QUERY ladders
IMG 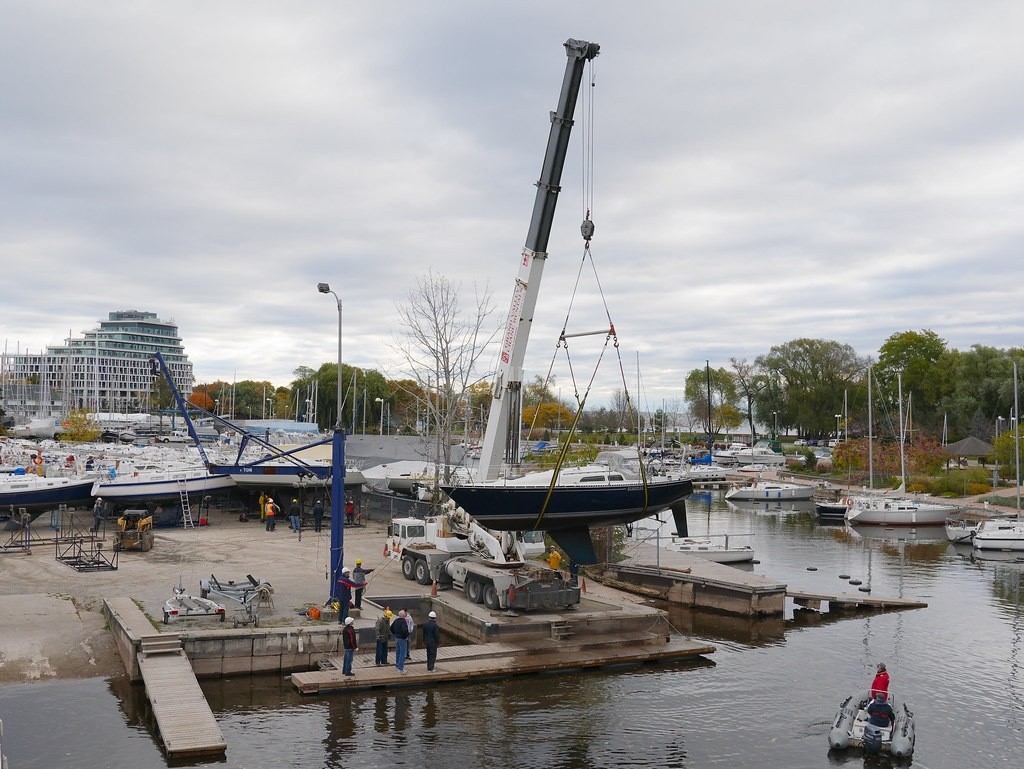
[177,476,194,530]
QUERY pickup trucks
[159,431,194,443]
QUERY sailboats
[815,355,1024,551]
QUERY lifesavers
[77,464,83,473]
[959,521,964,529]
[34,455,43,464]
[847,499,853,505]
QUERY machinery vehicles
[385,39,648,613]
[112,510,155,552]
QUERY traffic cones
[582,577,588,596]
[429,578,440,598]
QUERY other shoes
[406,657,412,660]
[381,661,390,664]
[342,671,345,674]
[345,672,355,676]
[376,662,381,666]
[428,669,437,673]
[395,667,406,675]
[342,622,344,625]
[338,621,341,624]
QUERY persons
[375,610,393,666]
[423,610,440,673]
[336,567,367,628]
[867,693,895,728]
[352,558,375,610]
[342,617,359,676]
[345,498,358,523]
[389,609,409,672]
[265,427,272,443]
[93,497,104,531]
[287,499,302,533]
[224,433,231,445]
[265,498,281,531]
[870,663,889,702]
[401,608,414,661]
[86,456,94,471]
[313,500,325,532]
[259,490,268,523]
[544,545,562,571]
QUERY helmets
[293,499,297,503]
[98,498,102,501]
[385,610,393,618]
[269,499,273,502]
[345,617,354,625]
[342,567,349,573]
[429,611,436,618]
[317,501,321,503]
[356,559,362,564]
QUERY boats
[630,351,786,480]
[410,434,693,528]
[828,689,916,757]
[0,329,475,513]
[637,532,756,562]
[725,480,815,500]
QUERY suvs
[794,438,844,448]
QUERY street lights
[835,414,841,444]
[318,283,342,429]
[266,398,271,420]
[285,406,288,420]
[375,398,383,436]
[999,418,1005,439]
[774,411,777,439]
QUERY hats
[876,693,884,698]
[549,546,556,549]
